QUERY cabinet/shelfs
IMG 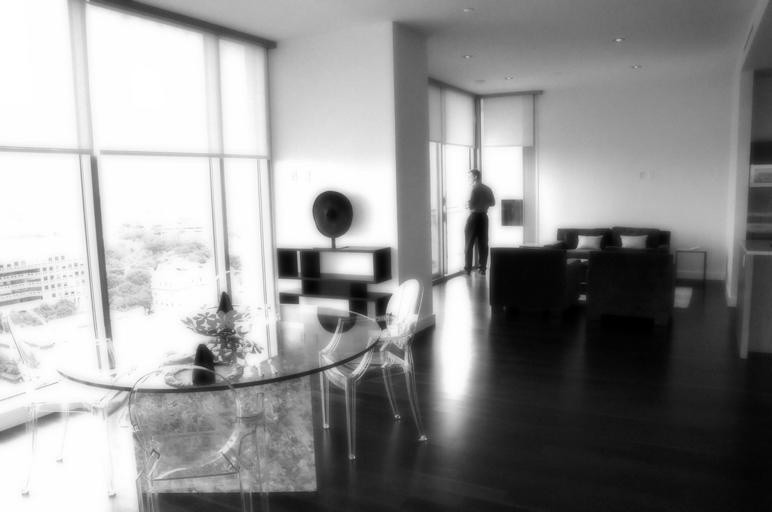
[276,246,392,334]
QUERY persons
[460,168,496,275]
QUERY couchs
[543,228,672,258]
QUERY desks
[674,247,708,302]
[737,238,772,360]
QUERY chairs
[0,280,427,511]
[490,247,673,325]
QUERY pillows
[575,235,648,252]
